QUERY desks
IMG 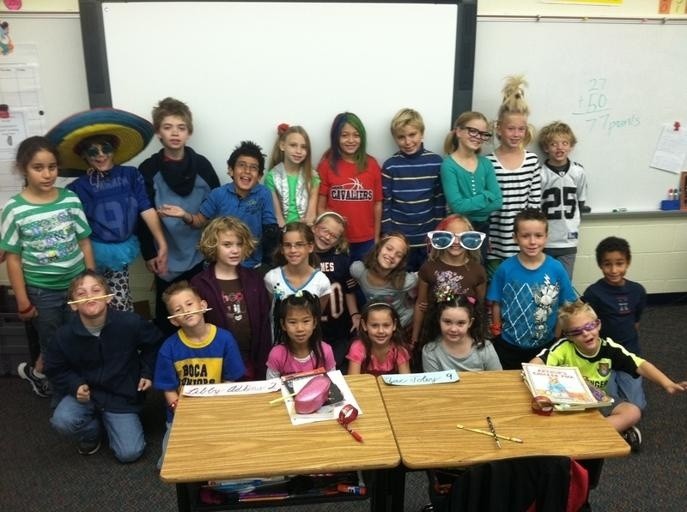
[375,361,633,512]
[155,369,405,511]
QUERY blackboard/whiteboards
[471,15,687,220]
[0,10,92,224]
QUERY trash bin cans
[0,314,30,377]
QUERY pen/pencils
[612,207,628,213]
[457,416,524,449]
[668,188,682,200]
[239,485,366,502]
[167,308,214,319]
[67,294,116,304]
[270,391,336,404]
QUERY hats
[43,107,154,177]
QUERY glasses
[316,223,337,240]
[460,126,492,141]
[427,230,487,251]
[86,145,114,158]
[563,319,600,337]
[279,241,311,250]
[234,161,260,171]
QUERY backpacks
[422,454,597,512]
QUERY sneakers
[621,425,642,452]
[17,361,54,398]
[76,433,102,456]
